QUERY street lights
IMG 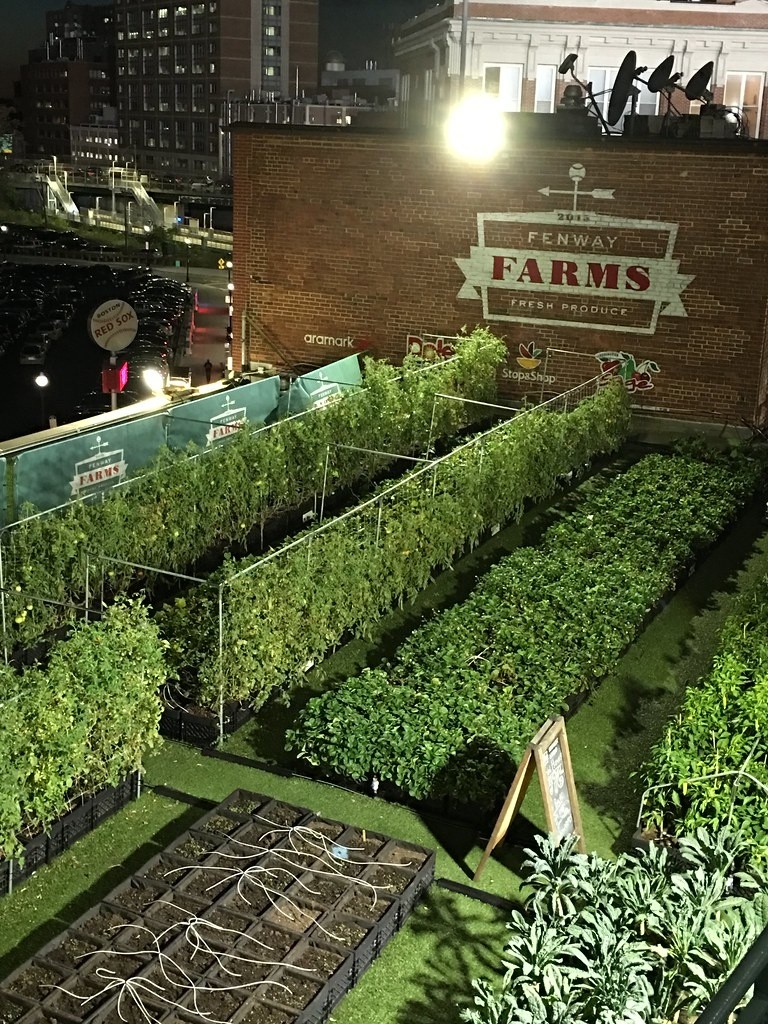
[51,155,57,175]
[96,197,103,218]
[227,283,234,305]
[225,260,233,282]
[209,207,216,230]
[35,372,49,431]
[228,306,234,327]
[125,161,131,191]
[127,201,134,225]
[203,212,210,229]
[112,160,119,189]
[174,201,182,225]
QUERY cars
[0,220,195,429]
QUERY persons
[216,362,224,378]
[203,359,212,384]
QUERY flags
[0,134,12,153]
